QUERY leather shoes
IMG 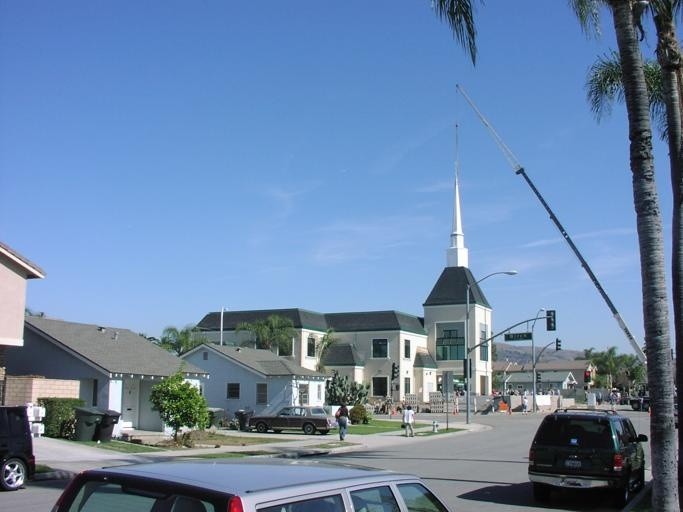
[406,435,414,437]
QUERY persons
[523,396,529,415]
[611,387,620,411]
[403,406,415,436]
[336,402,351,441]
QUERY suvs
[50,460,448,512]
[526,407,647,505]
[0,405,36,489]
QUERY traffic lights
[555,338,560,351]
[583,371,590,383]
[391,362,398,380]
[535,372,541,384]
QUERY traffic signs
[504,333,531,341]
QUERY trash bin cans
[93,410,122,442]
[235,411,255,432]
[74,407,105,441]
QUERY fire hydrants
[431,421,439,434]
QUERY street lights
[502,361,516,395]
[464,270,517,425]
[531,308,545,414]
[219,305,230,345]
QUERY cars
[589,388,629,406]
[248,406,337,435]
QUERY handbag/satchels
[401,424,406,428]
[335,410,341,417]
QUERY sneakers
[340,435,344,440]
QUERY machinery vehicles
[629,380,678,413]
